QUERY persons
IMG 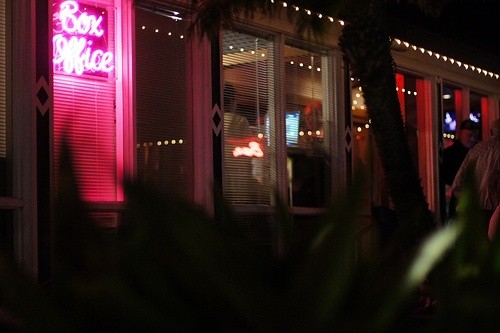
[221,80,256,203]
[439,119,483,218]
[444,119,500,220]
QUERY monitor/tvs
[264,109,312,149]
